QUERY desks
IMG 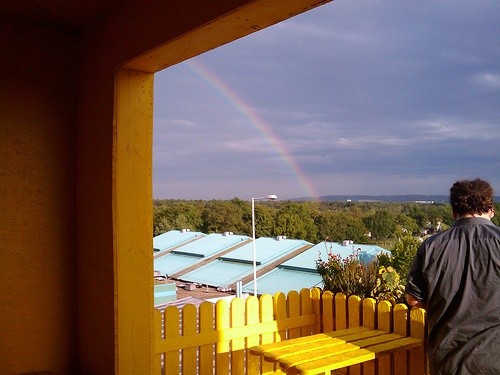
[250,324,423,375]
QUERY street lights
[251,194,277,297]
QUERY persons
[403,176,500,375]
